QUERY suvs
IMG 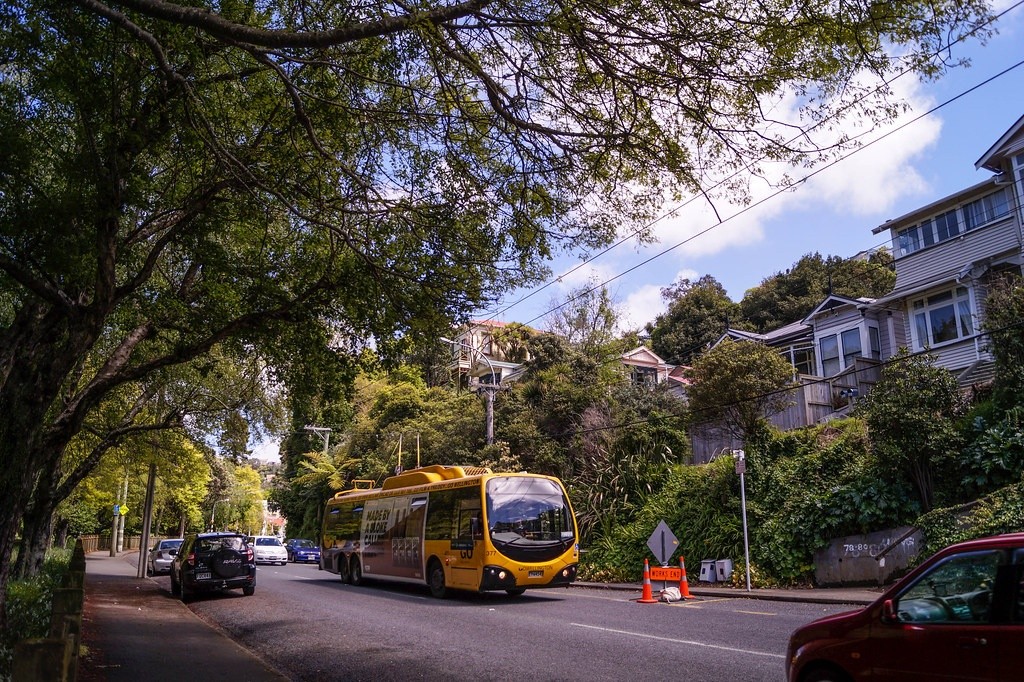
[170,531,256,601]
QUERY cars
[245,535,288,567]
[785,532,1024,682]
[148,538,188,575]
[285,539,320,564]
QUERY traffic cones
[637,558,659,603]
[675,556,695,598]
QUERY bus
[319,465,580,598]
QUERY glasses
[479,516,481,519]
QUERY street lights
[211,499,229,531]
[439,337,495,465]
